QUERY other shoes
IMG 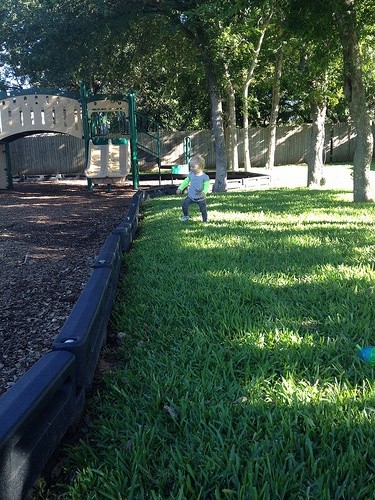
[181,215,189,221]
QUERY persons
[175,155,211,226]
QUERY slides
[82,138,132,183]
